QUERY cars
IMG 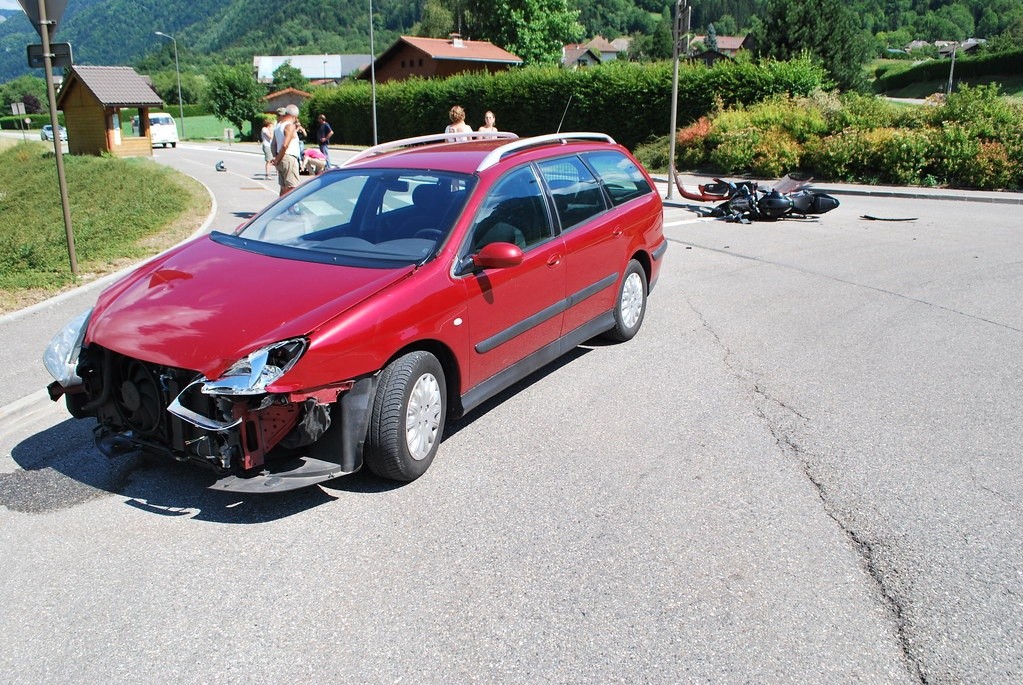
[41,128,667,493]
[41,125,69,144]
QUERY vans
[132,111,181,151]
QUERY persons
[445,106,473,191]
[261,104,334,216]
[476,111,497,140]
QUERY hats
[276,107,286,116]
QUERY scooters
[685,170,841,224]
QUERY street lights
[153,30,185,136]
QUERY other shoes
[266,174,273,180]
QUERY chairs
[469,184,526,254]
[394,184,454,239]
